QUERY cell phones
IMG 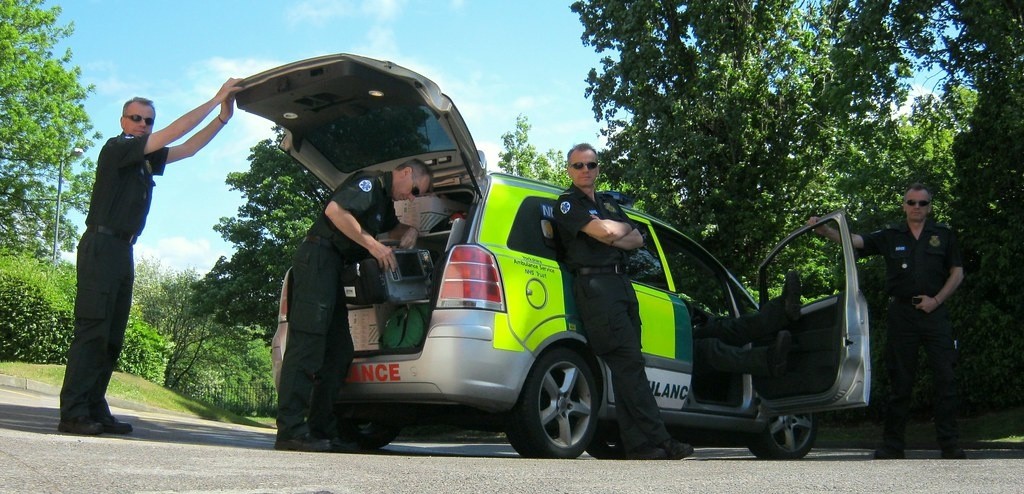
[912,297,922,305]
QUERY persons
[553,143,694,461]
[680,270,802,379]
[807,182,967,460]
[275,158,434,453]
[58,78,243,437]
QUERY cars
[230,52,870,461]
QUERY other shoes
[767,330,791,377]
[627,442,665,460]
[661,440,694,460]
[941,447,966,458]
[775,272,801,320]
[874,446,905,459]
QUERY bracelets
[408,226,420,234]
[609,240,616,248]
[218,114,228,125]
[935,296,941,307]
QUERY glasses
[411,177,420,197]
[906,200,929,206]
[124,115,154,125]
[571,162,597,170]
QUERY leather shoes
[92,412,133,433]
[276,436,332,451]
[59,416,103,434]
[320,435,358,453]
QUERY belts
[303,235,342,256]
[576,266,629,275]
[87,224,136,245]
[892,295,922,306]
